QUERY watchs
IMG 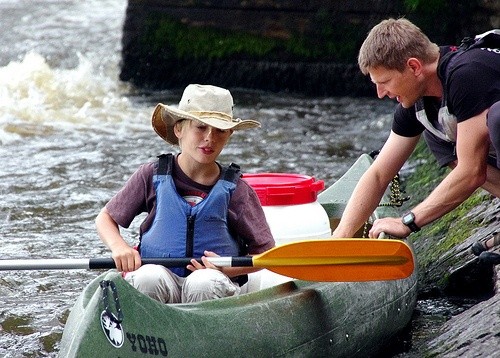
[401,212,421,234]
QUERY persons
[96,84,274,303]
[333,18,500,256]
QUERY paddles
[0,238,414,282]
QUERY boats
[58,153,418,358]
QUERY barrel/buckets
[240,173,333,288]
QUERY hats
[151,83,262,134]
